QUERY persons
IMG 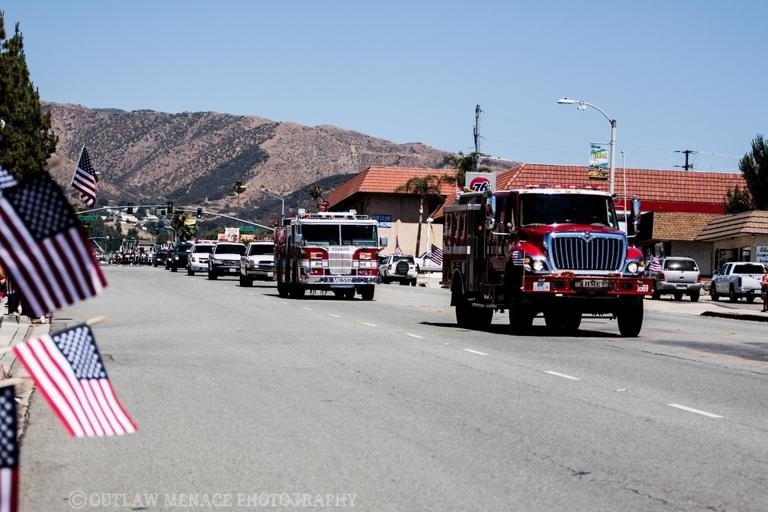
[759,266,768,311]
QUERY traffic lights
[160,204,166,216]
[195,206,204,221]
[166,202,174,215]
[125,202,135,215]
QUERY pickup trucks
[706,260,767,305]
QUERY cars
[412,248,444,274]
[94,247,173,267]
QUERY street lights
[556,96,616,205]
[238,182,288,228]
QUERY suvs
[235,238,279,288]
[168,240,193,275]
[375,252,421,288]
[183,242,218,276]
[639,253,705,305]
[205,240,244,280]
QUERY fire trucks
[266,205,388,301]
[439,181,654,338]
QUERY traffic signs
[78,216,96,221]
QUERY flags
[511,251,523,266]
[0,386,21,512]
[429,244,443,265]
[14,324,140,437]
[395,243,403,255]
[649,257,663,272]
[73,147,99,208]
[0,170,107,318]
